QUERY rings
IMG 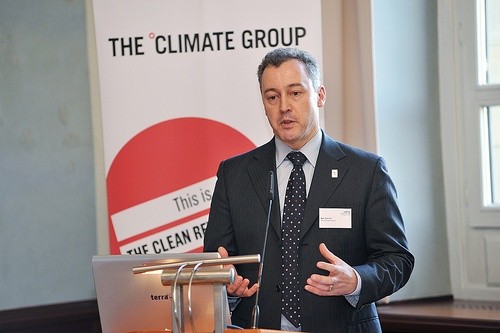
[329,284,333,291]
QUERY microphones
[250,171,275,329]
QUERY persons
[204,47,415,333]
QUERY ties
[280,152,307,328]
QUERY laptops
[91,252,232,333]
